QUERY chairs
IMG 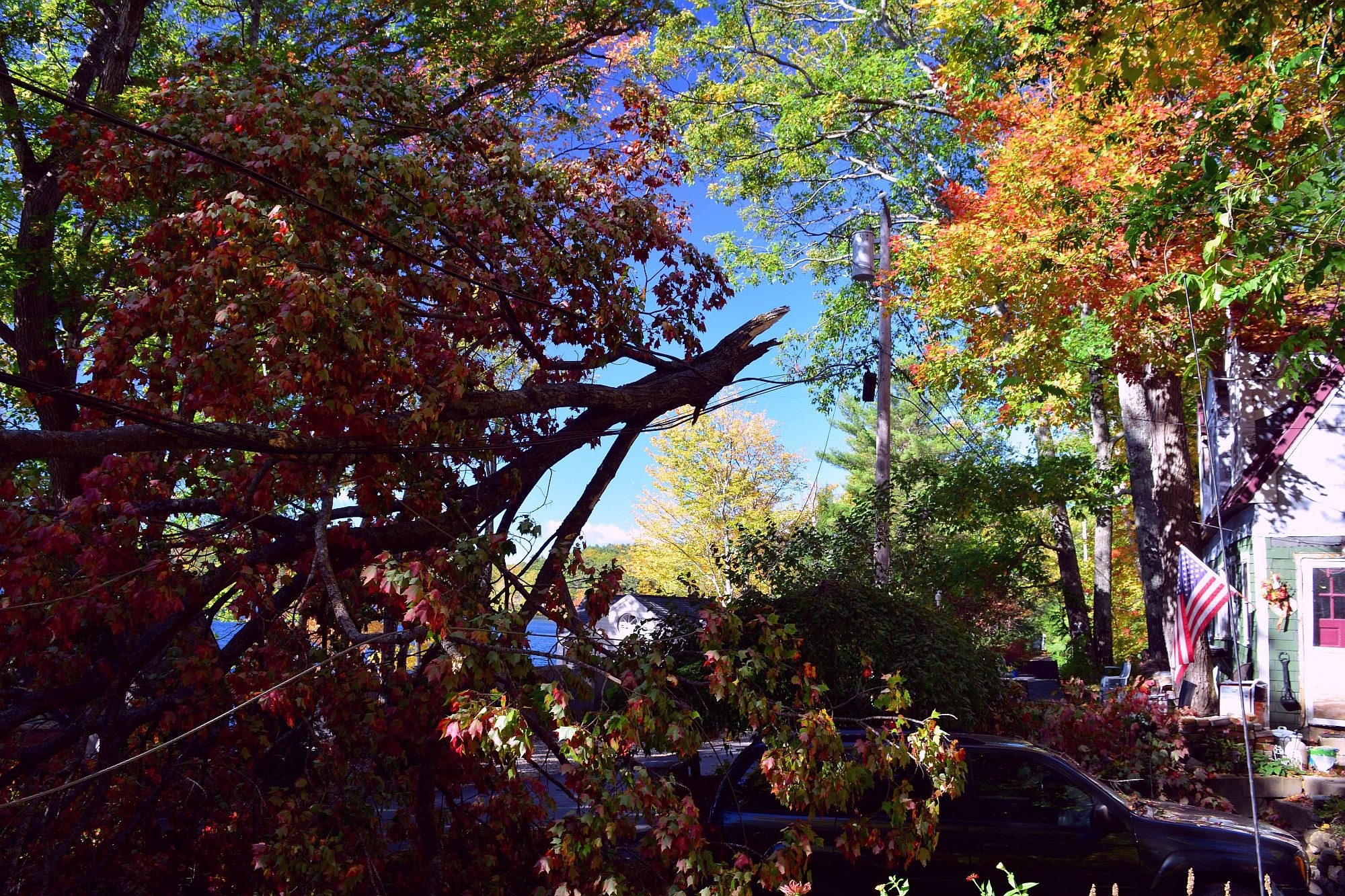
[1148,679,1197,713]
[1027,660,1072,702]
[1101,661,1132,701]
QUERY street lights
[852,210,892,593]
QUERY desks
[1000,677,1061,702]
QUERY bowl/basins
[1309,749,1336,771]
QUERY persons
[1138,648,1155,669]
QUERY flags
[1171,542,1237,690]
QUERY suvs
[707,732,1314,896]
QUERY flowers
[1261,573,1292,610]
